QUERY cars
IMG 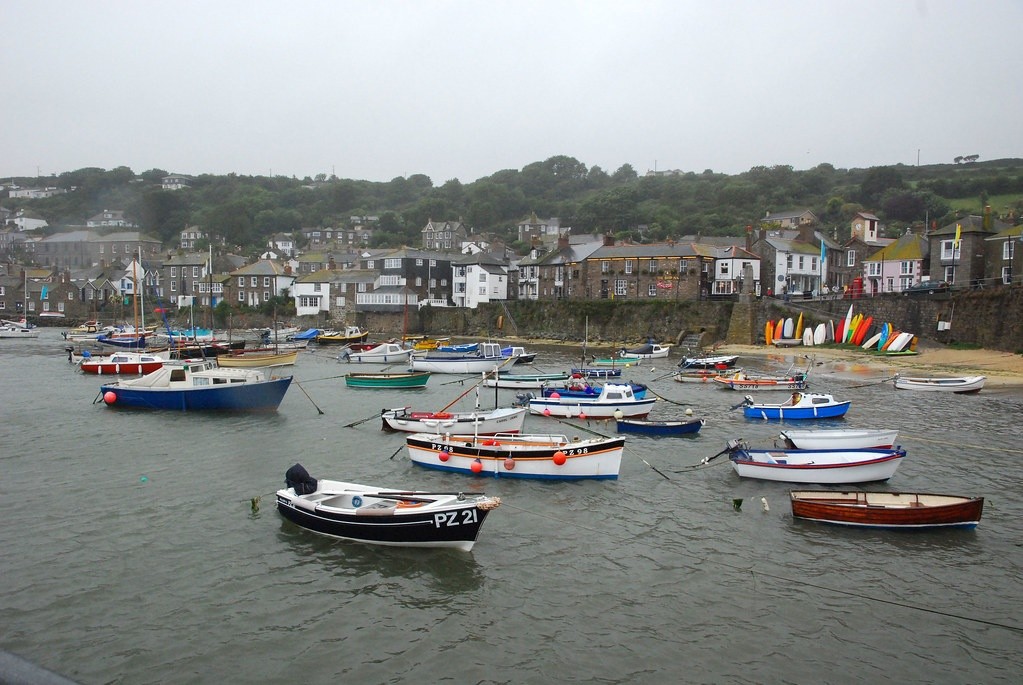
[901,280,949,296]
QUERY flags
[954,224,961,248]
[820,240,826,262]
[40,286,48,301]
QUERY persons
[798,372,810,388]
[768,287,772,296]
[264,328,270,345]
[812,288,818,301]
[345,347,352,363]
[822,285,829,299]
[832,283,839,299]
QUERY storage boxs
[429,414,454,419]
[411,411,432,419]
[775,460,786,464]
[397,500,423,508]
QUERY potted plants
[671,268,677,276]
[657,268,664,276]
[679,271,686,278]
[632,270,639,276]
[642,269,648,276]
[609,269,615,276]
[618,268,625,276]
[689,268,696,276]
[650,271,657,277]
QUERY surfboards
[795,312,803,338]
[862,322,901,351]
[826,303,873,346]
[765,317,784,345]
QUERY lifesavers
[567,373,585,391]
[482,439,500,446]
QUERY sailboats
[2,244,300,374]
[571,315,622,378]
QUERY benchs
[910,501,924,507]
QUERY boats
[540,375,647,400]
[780,429,900,449]
[482,372,569,389]
[788,488,984,530]
[894,373,986,394]
[275,463,502,551]
[100,362,293,412]
[515,391,657,418]
[764,304,919,356]
[0,328,40,338]
[615,418,706,435]
[248,327,537,373]
[673,355,852,420]
[381,364,625,481]
[726,438,907,484]
[344,372,431,387]
[588,343,670,366]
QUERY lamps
[537,275,542,280]
[563,271,567,277]
